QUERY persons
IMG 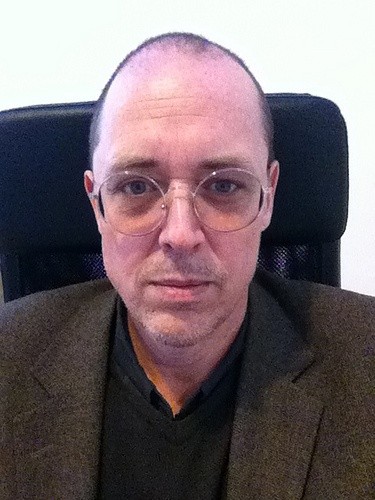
[0,33,373,500]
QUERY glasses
[80,164,275,235]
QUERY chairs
[0,93,349,303]
